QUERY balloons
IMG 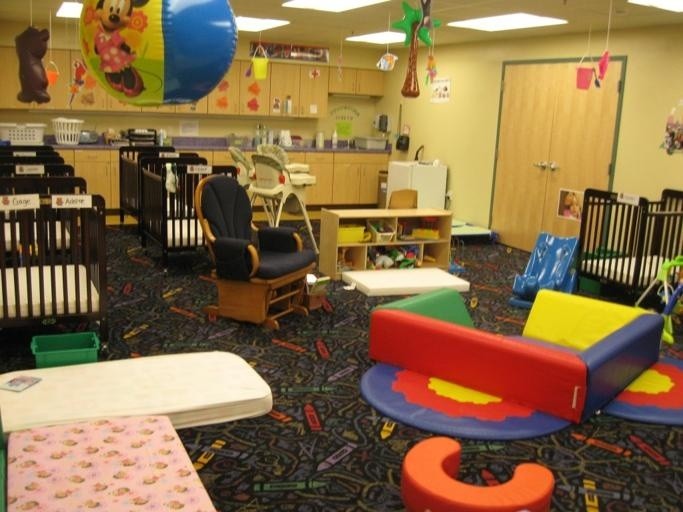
[15,26,51,102]
[391,2,441,97]
[79,0,238,106]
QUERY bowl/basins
[292,140,311,148]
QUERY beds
[572,188,683,314]
[119,146,237,273]
[0,141,107,368]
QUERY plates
[279,145,294,148]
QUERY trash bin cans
[378,170,388,209]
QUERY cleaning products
[254,122,267,145]
[332,130,338,148]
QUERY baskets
[50,117,85,146]
[0,121,50,147]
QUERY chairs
[245,151,321,259]
[254,142,312,221]
[225,143,274,229]
[192,172,320,330]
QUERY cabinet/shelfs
[0,45,387,118]
[236,151,389,209]
[319,207,453,282]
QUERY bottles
[373,112,388,132]
[158,129,171,146]
[315,133,323,148]
[331,130,337,149]
[286,95,293,113]
[251,120,273,147]
[102,129,130,148]
[227,132,251,147]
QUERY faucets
[414,145,424,160]
